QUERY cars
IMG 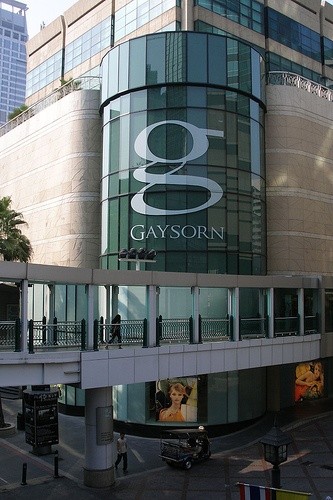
[158,428,212,471]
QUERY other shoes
[124,470,128,473]
[105,346,108,350]
[119,346,123,349]
[115,464,118,469]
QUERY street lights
[258,417,294,500]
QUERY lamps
[117,247,157,261]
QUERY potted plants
[9,76,87,130]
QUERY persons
[106,314,122,346]
[115,431,129,473]
[294,363,322,401]
[157,382,186,423]
